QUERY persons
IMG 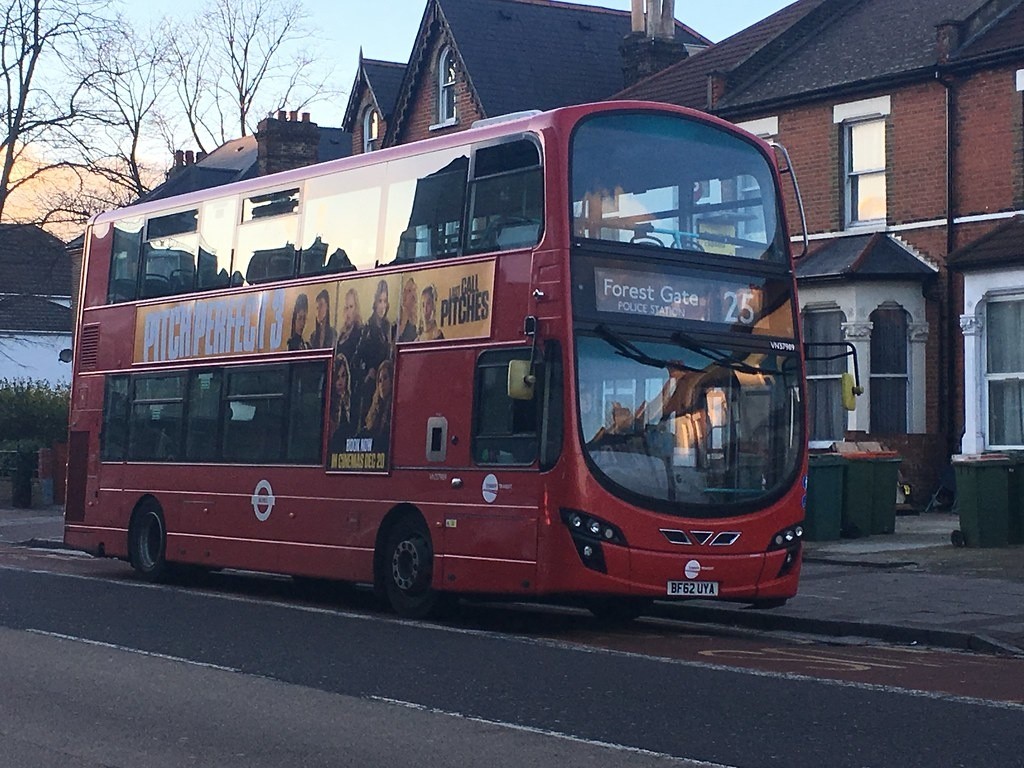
[328,353,352,450]
[390,277,420,342]
[336,288,364,363]
[286,293,311,351]
[310,289,338,349]
[360,280,392,371]
[413,283,445,342]
[365,360,392,441]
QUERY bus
[63,98,864,623]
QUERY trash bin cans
[950,455,1010,549]
[984,450,1024,545]
[801,454,847,543]
[841,452,873,539]
[869,453,903,536]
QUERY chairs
[111,214,527,303]
